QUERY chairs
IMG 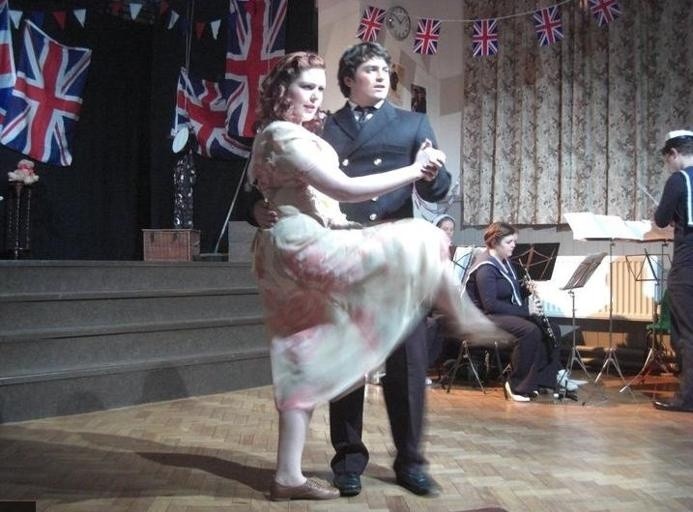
[446,340,507,400]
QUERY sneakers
[505,380,531,402]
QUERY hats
[660,129,693,150]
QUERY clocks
[387,7,410,40]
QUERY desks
[141,229,200,262]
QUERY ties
[355,105,375,129]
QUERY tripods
[548,288,682,409]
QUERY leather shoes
[455,324,517,347]
[396,468,443,498]
[334,473,362,496]
[271,474,340,501]
[652,399,693,412]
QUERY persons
[649,129,692,410]
[427,213,471,389]
[252,52,511,502]
[238,41,454,497]
[453,221,572,402]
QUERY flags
[472,19,498,58]
[0,19,93,166]
[588,1,620,28]
[410,16,443,57]
[355,5,385,43]
[172,2,288,162]
[532,5,565,48]
